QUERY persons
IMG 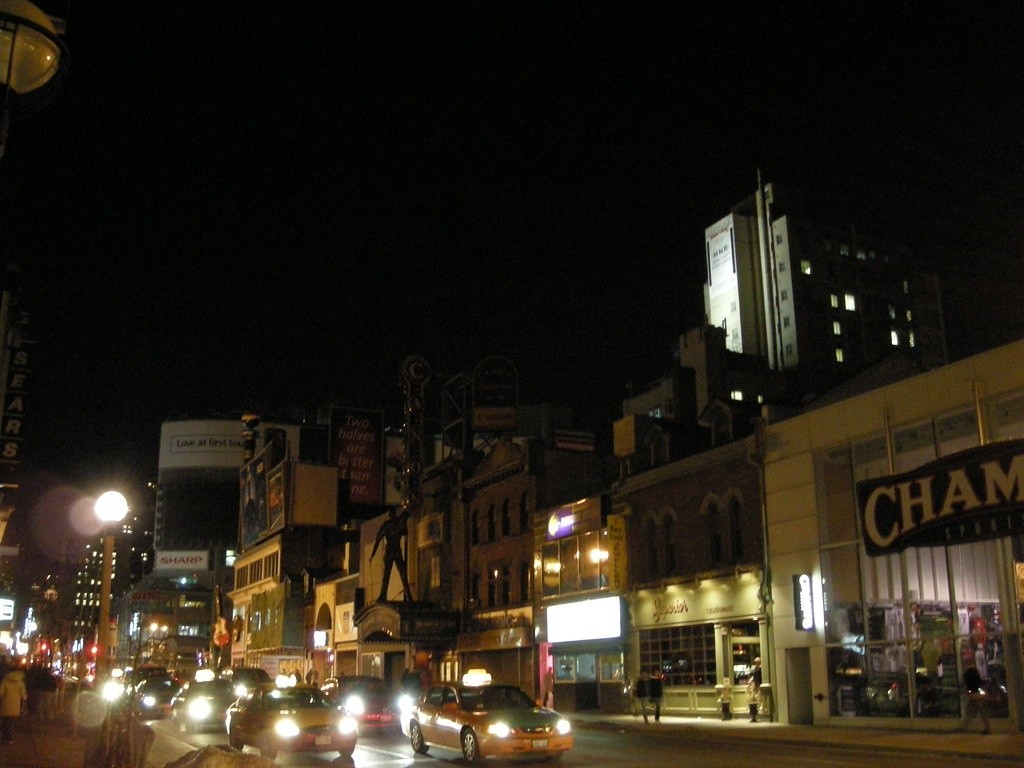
[717,678,732,721]
[636,668,663,724]
[745,675,761,722]
[370,507,413,603]
[0,655,57,745]
[495,685,516,709]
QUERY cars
[171,670,238,734]
[225,676,359,759]
[219,668,272,698]
[133,678,180,720]
[401,669,573,768]
[321,674,400,735]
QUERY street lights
[84,491,128,768]
